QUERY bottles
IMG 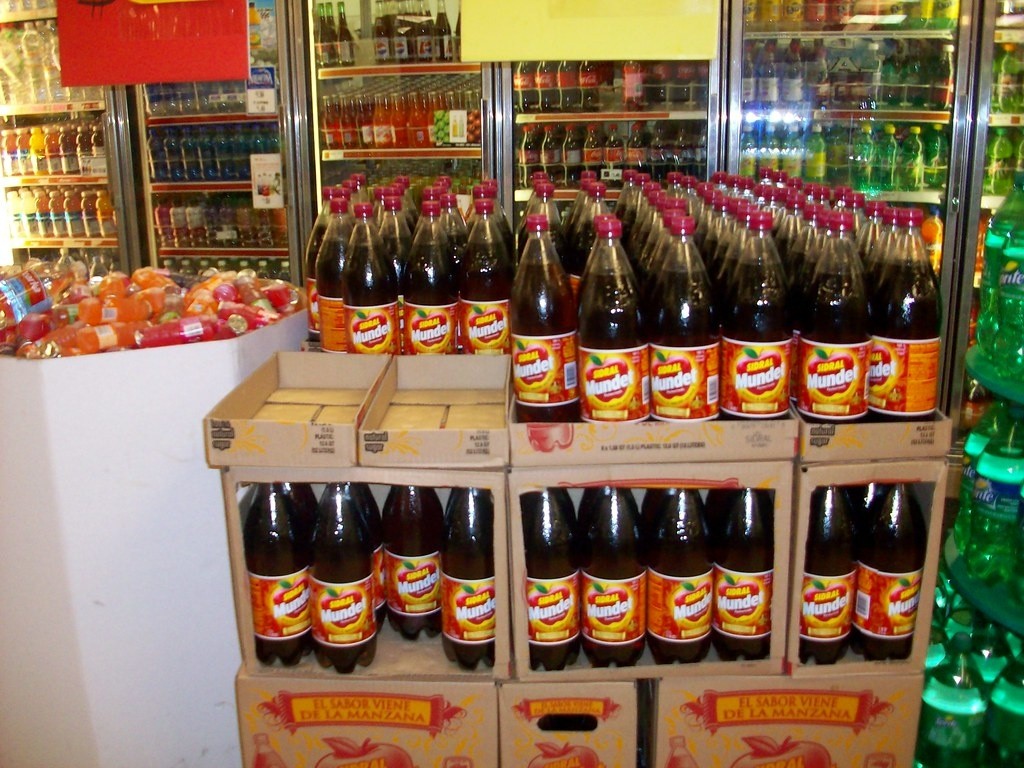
[0,0,307,358]
[244,482,313,668]
[313,0,463,68]
[275,482,318,566]
[307,0,1024,768]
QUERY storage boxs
[204,344,952,680]
[235,662,498,768]
[499,680,637,768]
[653,673,924,768]
